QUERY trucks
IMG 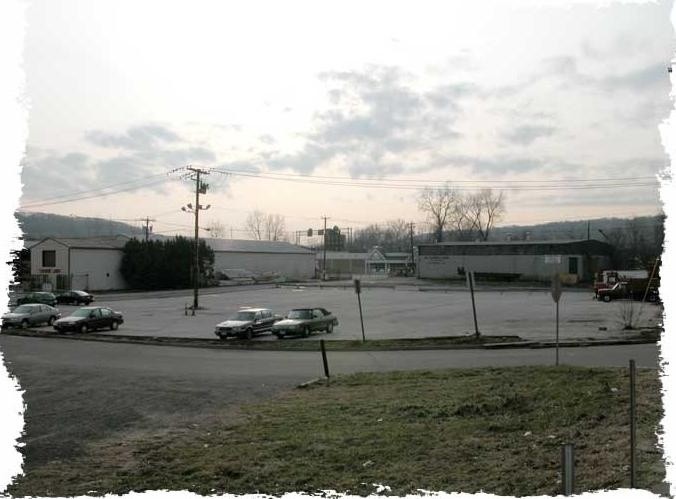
[597,278,659,302]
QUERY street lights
[181,203,211,309]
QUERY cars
[214,307,339,340]
[1,291,123,335]
